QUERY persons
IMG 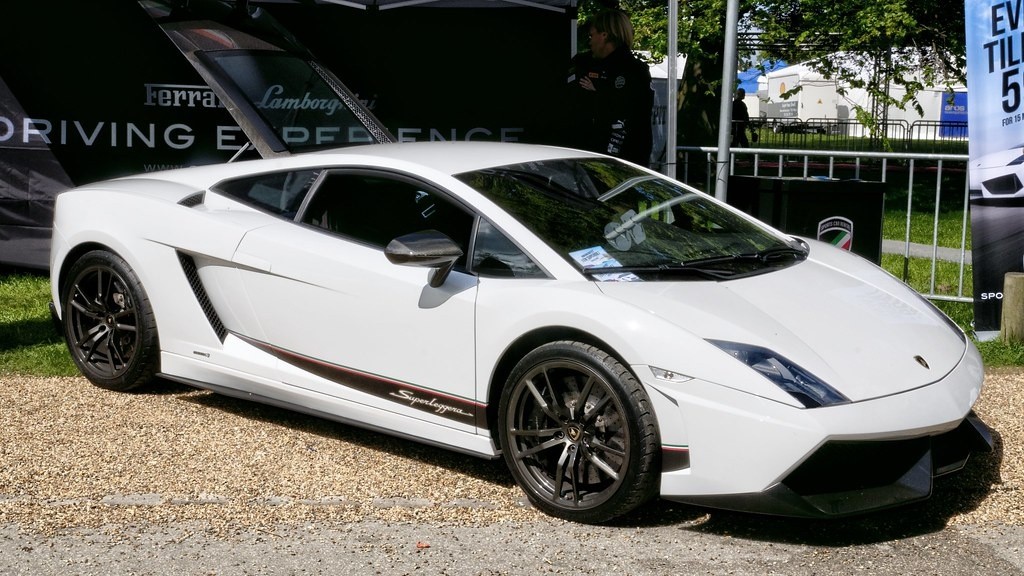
[558,5,655,170]
[730,88,757,161]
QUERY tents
[0,0,578,271]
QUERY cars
[969,137,1024,199]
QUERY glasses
[588,34,593,38]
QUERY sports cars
[46,17,999,529]
